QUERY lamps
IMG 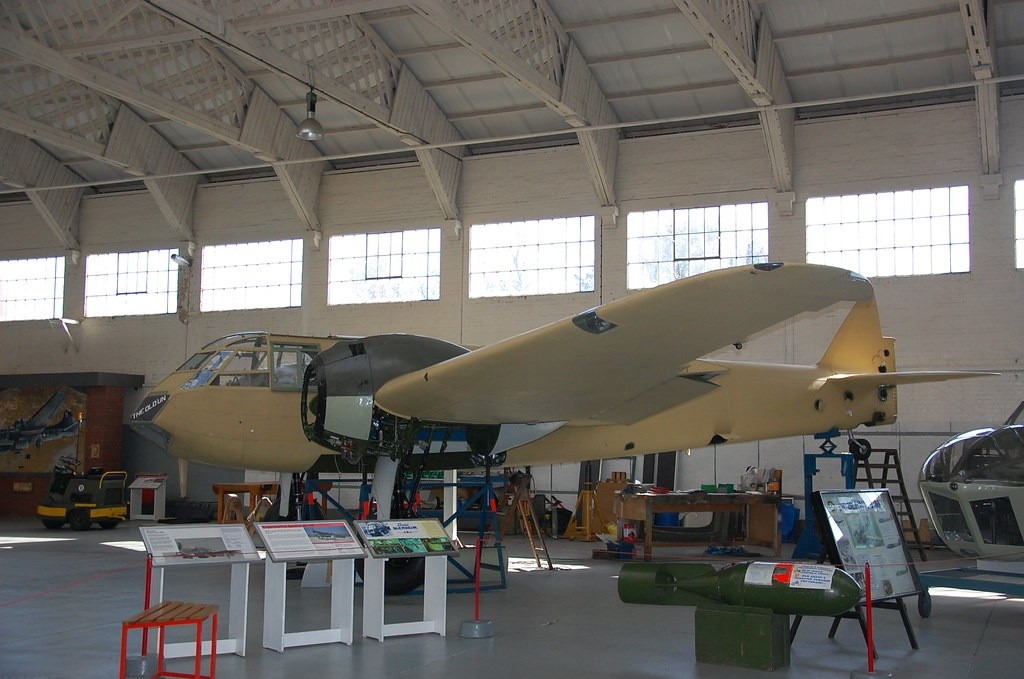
[296,90,324,140]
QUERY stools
[120,601,220,679]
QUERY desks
[212,482,332,524]
[622,493,782,562]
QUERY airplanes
[128,264,1003,595]
[918,398,1024,563]
